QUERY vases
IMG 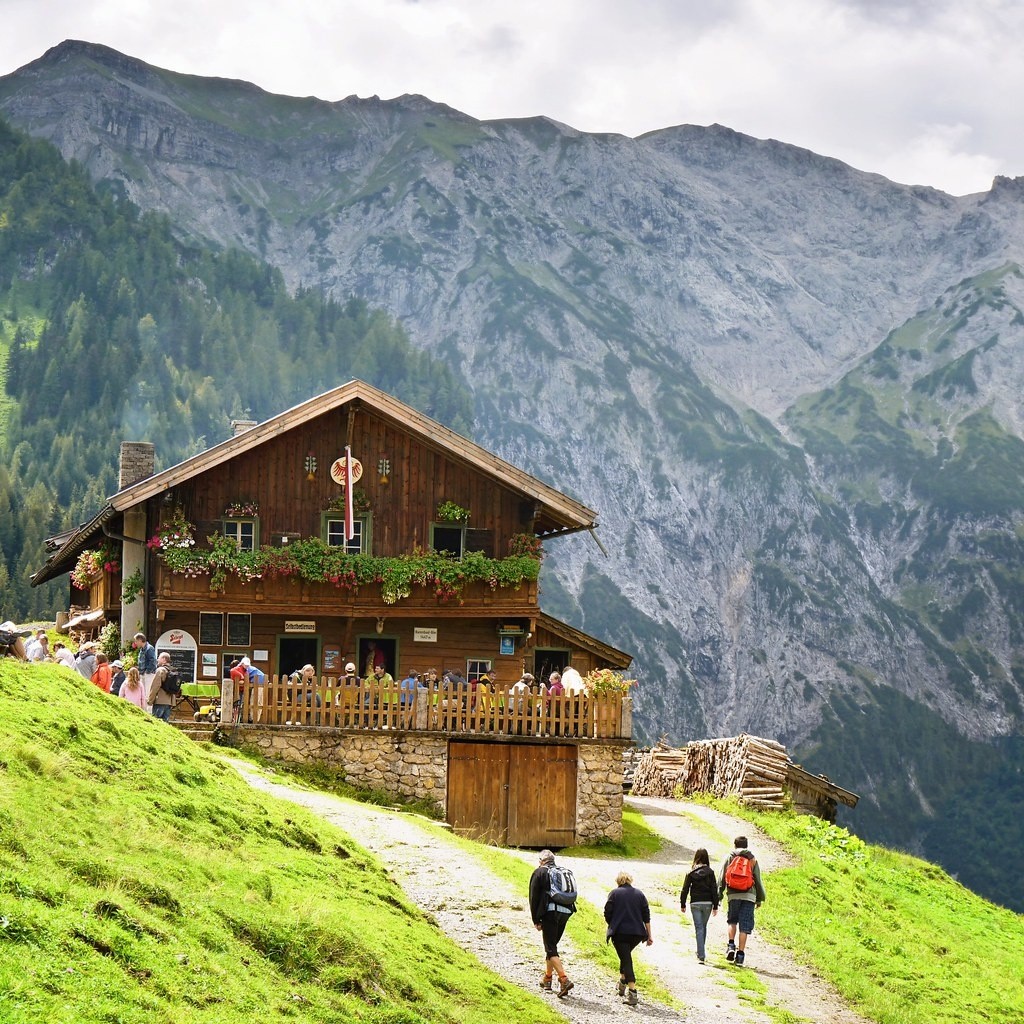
[330,504,365,511]
[594,697,631,739]
[226,510,258,516]
[436,513,466,522]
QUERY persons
[716,837,766,967]
[529,850,574,998]
[604,868,653,1005]
[0,620,176,721]
[230,657,589,737]
[680,849,718,965]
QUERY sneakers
[616,980,627,994]
[622,990,637,1005]
[725,944,735,961]
[556,976,574,997]
[734,951,744,966]
[538,973,552,989]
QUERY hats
[111,660,123,668]
[734,836,749,848]
[442,669,451,676]
[40,634,49,644]
[345,662,355,671]
[241,657,250,665]
[83,642,97,650]
[539,850,555,861]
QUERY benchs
[278,700,588,732]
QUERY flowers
[582,666,639,698]
[224,499,260,519]
[67,539,123,591]
[328,488,371,511]
[147,507,549,606]
[116,640,141,670]
[436,501,472,530]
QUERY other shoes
[698,959,704,964]
[697,955,699,958]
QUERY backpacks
[160,665,182,694]
[539,865,577,906]
[724,852,756,892]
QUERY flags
[344,446,355,539]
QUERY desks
[180,683,220,711]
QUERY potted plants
[119,567,145,604]
